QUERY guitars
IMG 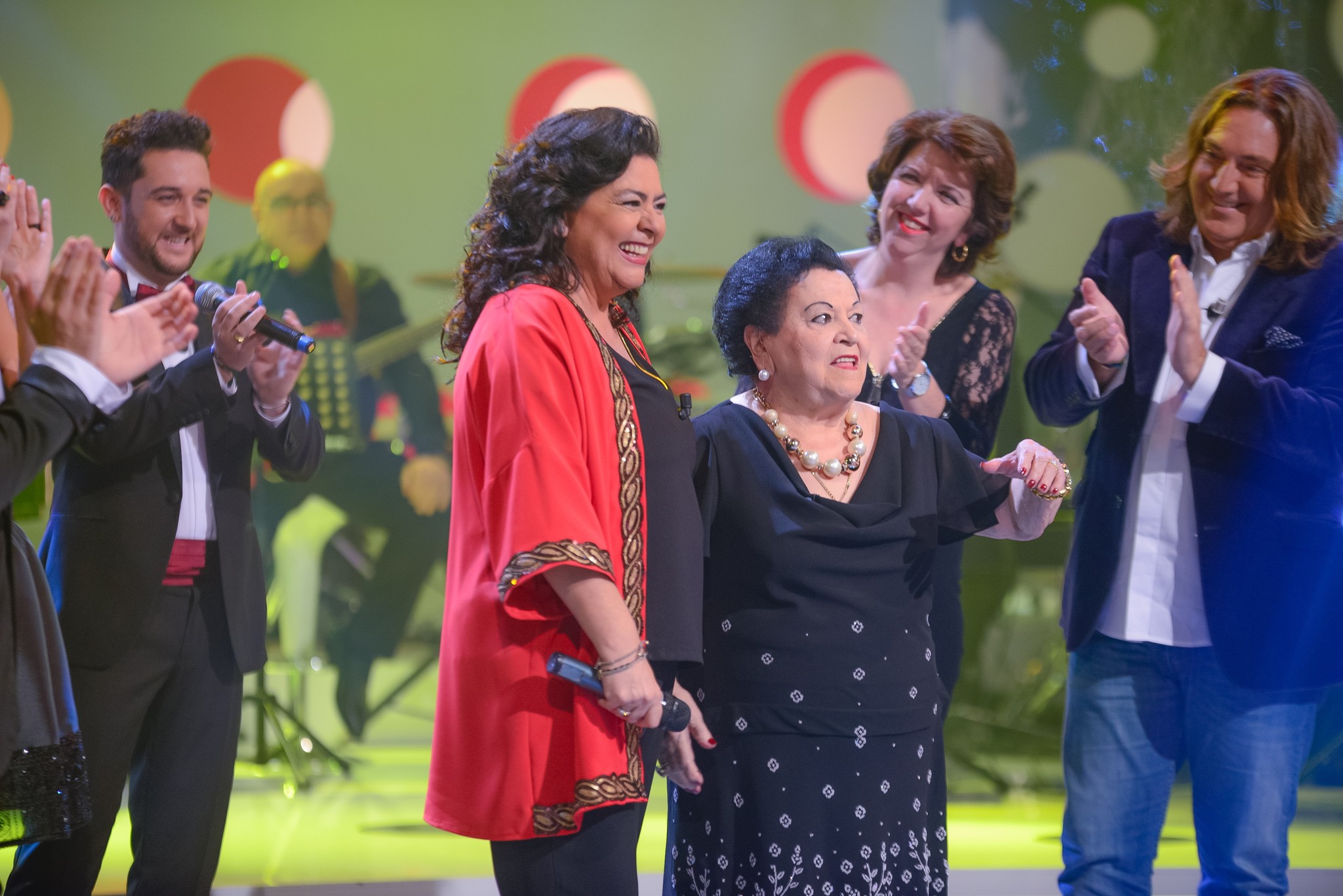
[357,310,451,381]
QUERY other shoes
[331,647,373,736]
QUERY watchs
[891,361,930,397]
[1024,459,1072,500]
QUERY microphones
[1208,300,1228,318]
[680,393,693,416]
[193,282,317,355]
[544,652,693,732]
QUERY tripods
[242,671,352,789]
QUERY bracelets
[256,398,288,409]
[1102,363,1122,368]
[594,641,649,680]
[211,344,240,376]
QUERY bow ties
[106,247,195,304]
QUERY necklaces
[753,386,866,502]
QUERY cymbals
[641,325,724,380]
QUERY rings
[1048,460,1059,470]
[233,336,244,344]
[656,763,670,777]
[29,223,41,230]
[618,706,631,716]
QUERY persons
[3,109,325,896]
[0,163,197,848]
[1024,68,1343,896]
[424,107,704,896]
[655,236,1072,896]
[736,112,1020,716]
[200,158,451,735]
